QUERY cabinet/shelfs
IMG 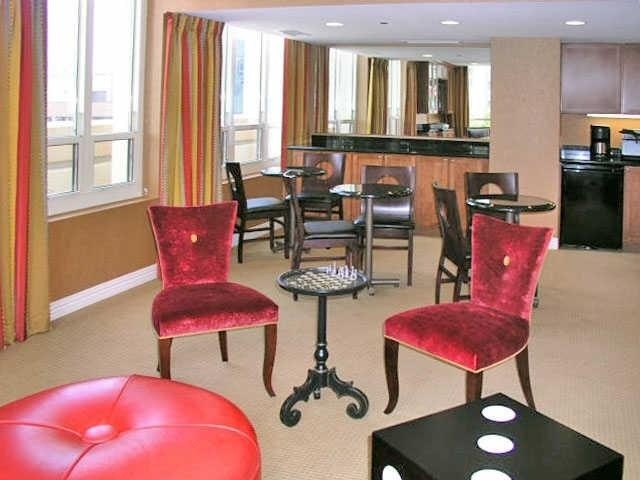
[288,147,353,229]
[348,150,417,221]
[620,45,640,116]
[562,40,622,115]
[413,157,487,239]
[622,165,639,247]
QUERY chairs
[145,199,280,397]
[426,182,471,305]
[468,124,489,138]
[353,164,418,287]
[220,161,296,263]
[295,152,348,252]
[381,210,552,411]
[462,169,521,226]
[279,168,365,302]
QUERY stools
[0,373,265,479]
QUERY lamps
[415,113,442,137]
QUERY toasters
[620,129,640,162]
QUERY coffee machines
[591,126,610,162]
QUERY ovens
[560,160,623,250]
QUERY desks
[258,165,330,255]
[273,264,370,428]
[329,181,416,301]
[459,193,557,311]
[370,389,627,479]
